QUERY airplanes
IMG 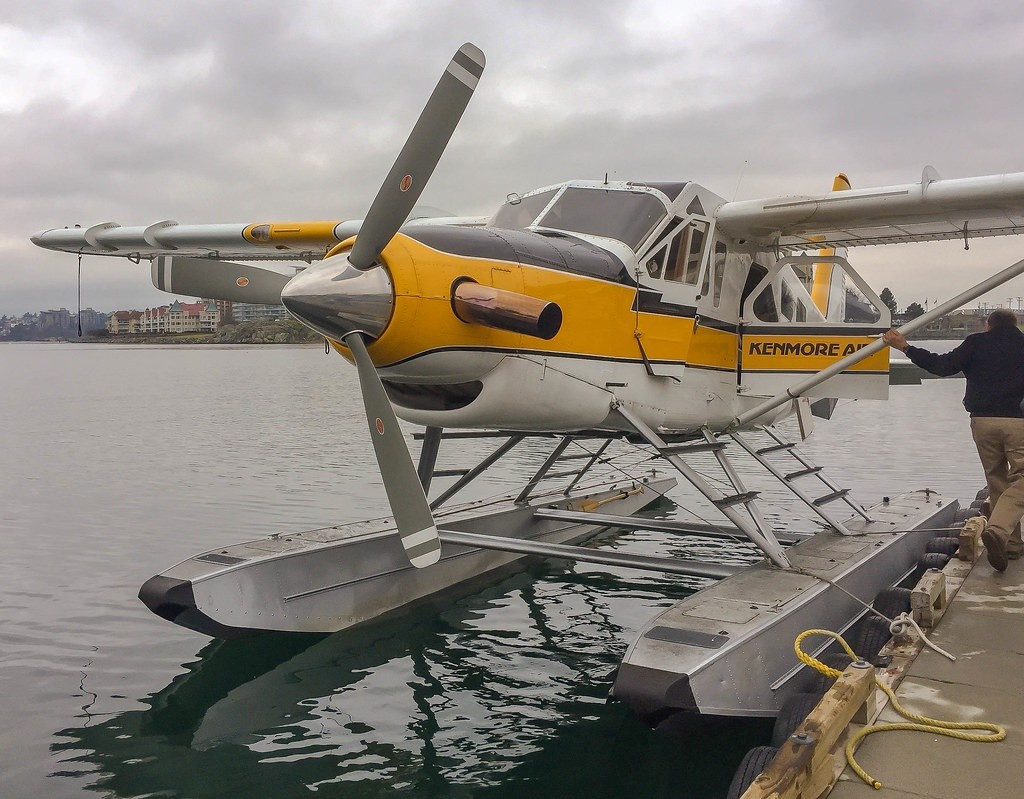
[30,44,1024,719]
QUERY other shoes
[981,529,1008,571]
[1006,549,1024,560]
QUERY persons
[882,309,1024,572]
